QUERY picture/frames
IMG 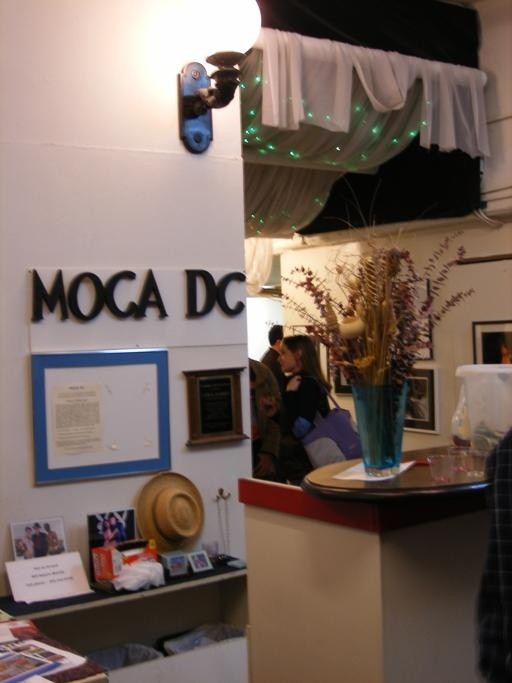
[473,319,511,364]
[405,278,433,361]
[333,364,437,435]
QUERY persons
[102,518,119,545]
[107,514,126,542]
[21,527,37,560]
[248,357,282,483]
[274,334,333,487]
[32,523,47,557]
[44,522,62,555]
[476,428,512,682]
[501,344,512,364]
[259,325,286,391]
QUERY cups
[426,443,488,483]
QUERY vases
[349,382,409,477]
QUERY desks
[1,554,249,656]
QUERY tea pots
[455,362,512,459]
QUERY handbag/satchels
[300,408,364,469]
